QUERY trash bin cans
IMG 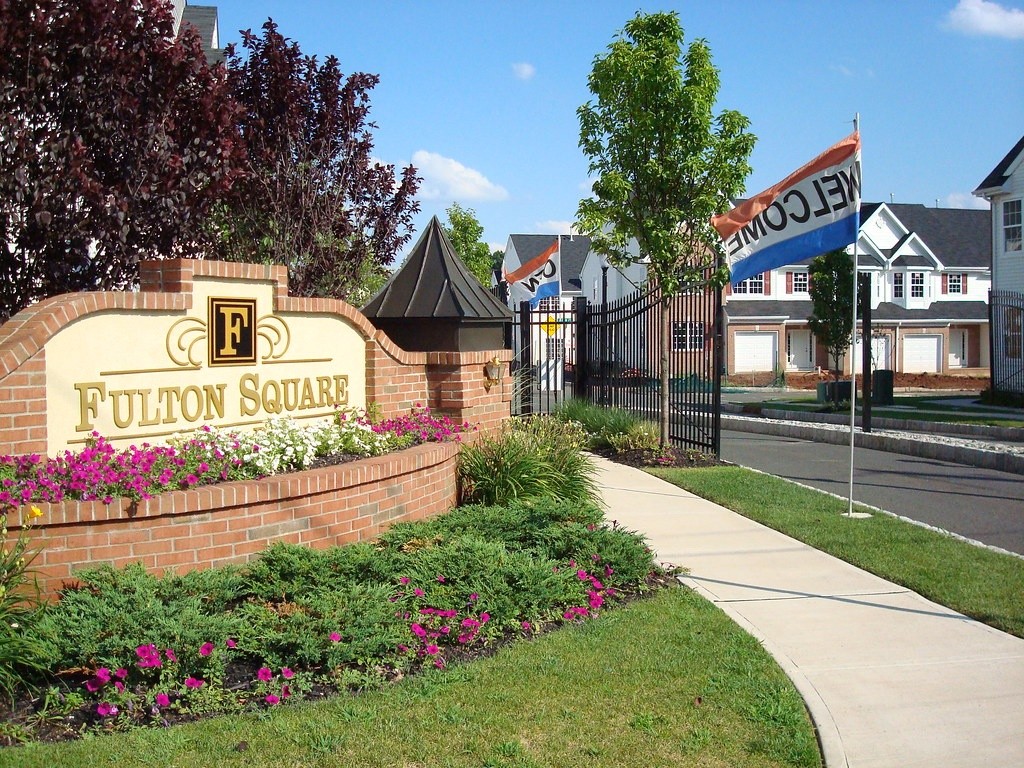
[815,381,857,402]
[872,369,894,404]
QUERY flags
[711,133,861,287]
[506,240,560,311]
